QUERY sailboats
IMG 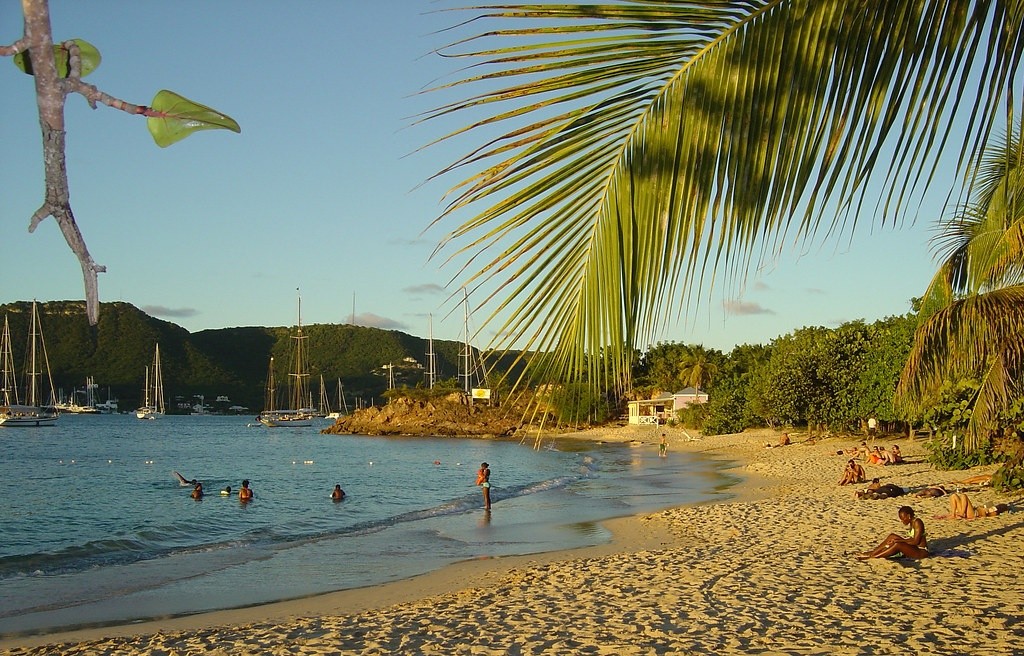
[42,375,103,414]
[260,295,316,426]
[136,343,166,419]
[0,298,59,428]
[261,356,316,414]
[309,373,350,419]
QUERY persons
[837,459,865,486]
[238,479,252,499]
[190,483,203,501]
[943,494,1000,520]
[480,462,491,510]
[778,433,791,445]
[873,484,911,500]
[658,433,669,458]
[913,485,956,500]
[853,478,880,500]
[847,442,902,466]
[855,506,929,563]
[866,414,877,443]
[331,484,345,499]
[225,486,231,494]
